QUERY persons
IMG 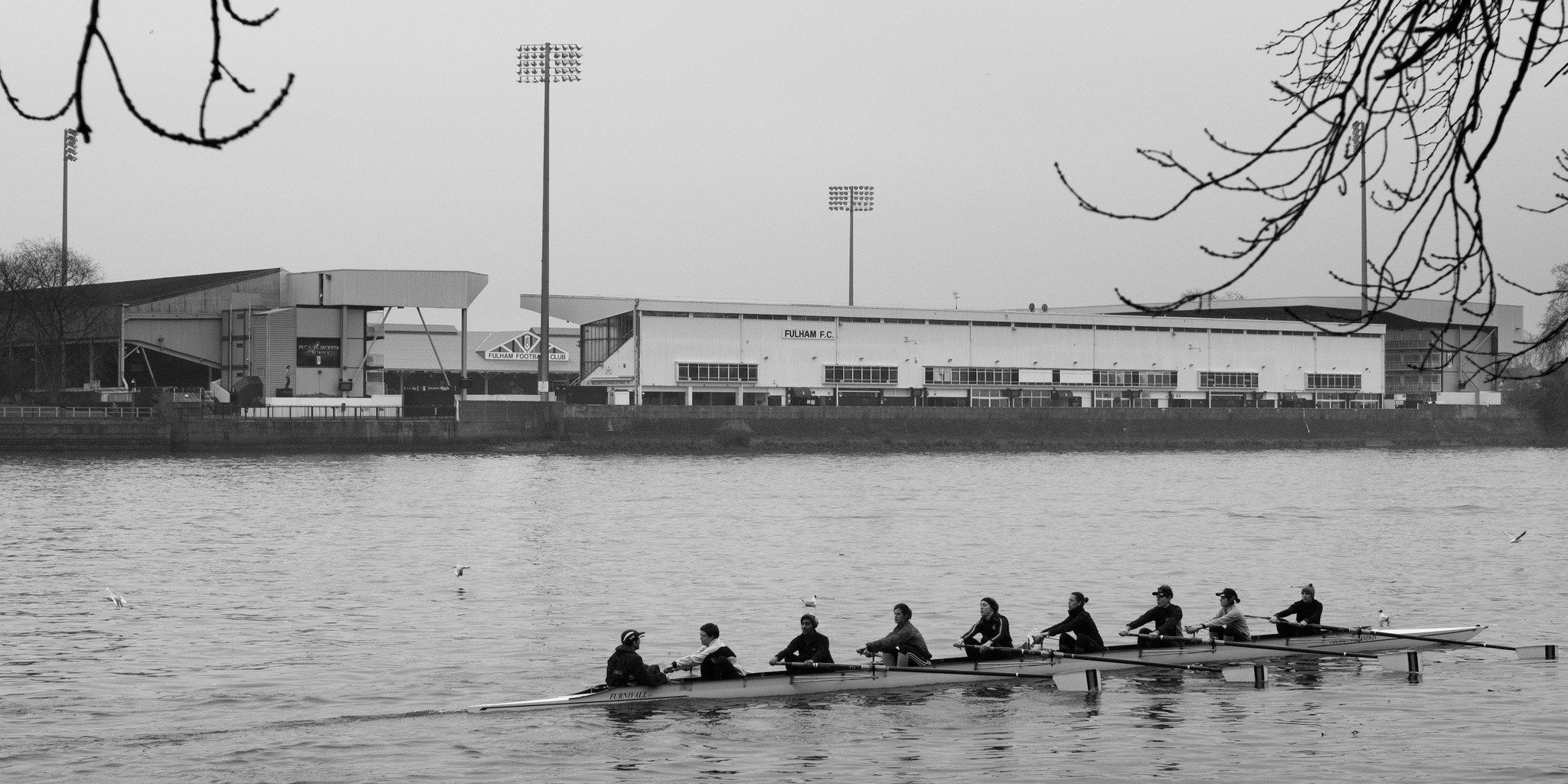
[605,629,669,689]
[1185,588,1252,645]
[1119,585,1184,649]
[856,603,932,671]
[663,623,746,682]
[1270,583,1323,634]
[284,366,294,389]
[769,613,835,676]
[954,598,1014,661]
[1033,592,1105,654]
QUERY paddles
[766,660,1104,693]
[951,641,1269,684]
[1245,613,1559,661]
[662,664,698,672]
[1121,632,1423,674]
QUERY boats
[468,623,1493,716]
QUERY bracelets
[1202,624,1206,628]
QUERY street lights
[1351,119,1369,325]
[516,41,583,402]
[60,127,79,286]
[829,185,874,306]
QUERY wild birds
[452,565,470,577]
[1503,530,1527,543]
[104,588,127,608]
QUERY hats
[1301,583,1315,598]
[801,613,819,627]
[621,629,646,642]
[1152,589,1173,598]
[1215,589,1236,601]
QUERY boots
[882,653,896,666]
[1223,634,1234,641]
[1209,632,1220,640]
[897,652,908,667]
